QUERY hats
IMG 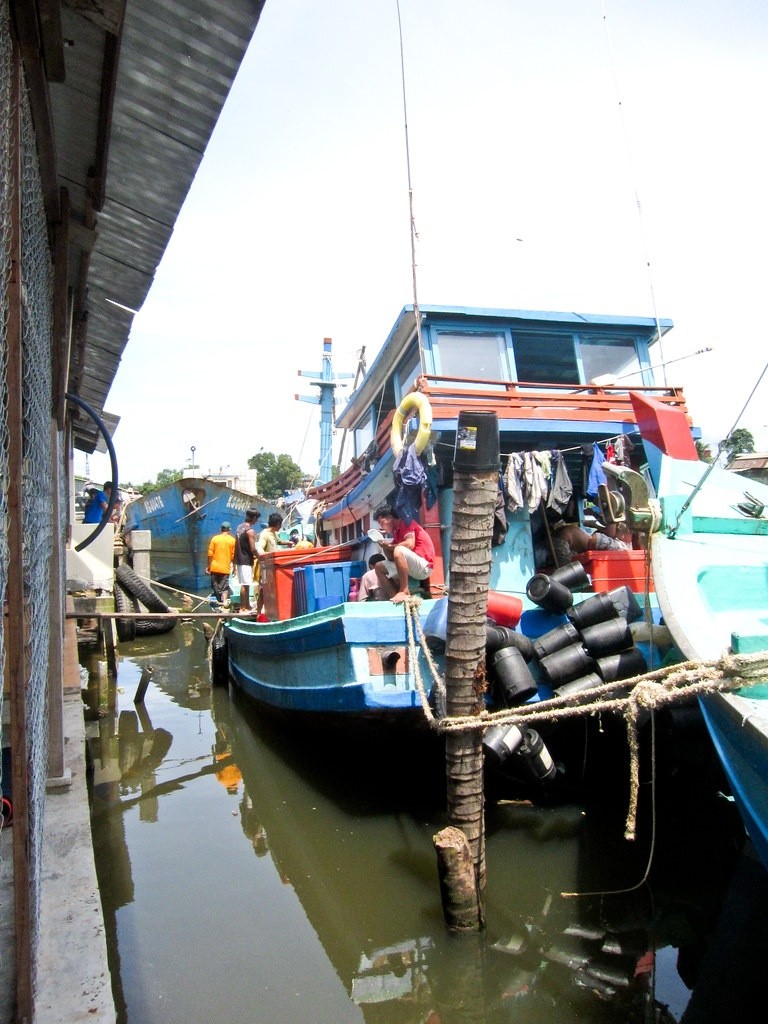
[85,482,90,485]
[221,522,231,530]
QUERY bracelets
[388,544,391,550]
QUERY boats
[598,390,768,868]
[210,300,704,709]
[120,475,287,590]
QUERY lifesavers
[389,390,434,458]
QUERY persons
[232,506,265,614]
[204,521,237,609]
[80,480,127,530]
[530,509,634,574]
[288,528,317,548]
[371,504,437,603]
[253,512,295,614]
[356,554,400,601]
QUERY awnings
[65,0,270,460]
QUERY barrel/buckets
[482,718,559,789]
[425,561,648,706]
